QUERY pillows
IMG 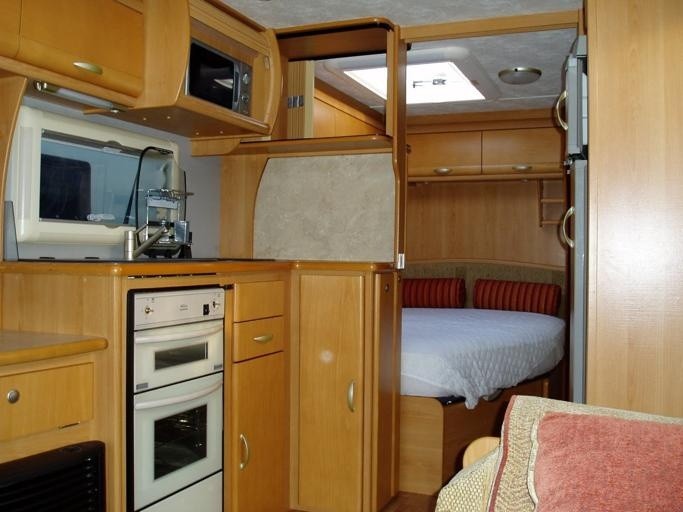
[469,277,562,318]
[524,406,681,512]
[398,275,469,310]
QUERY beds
[388,253,572,501]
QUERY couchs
[426,384,682,510]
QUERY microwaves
[183,35,253,118]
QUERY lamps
[319,46,501,111]
[496,66,542,87]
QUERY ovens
[131,288,224,510]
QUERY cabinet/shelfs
[223,279,291,509]
[0,0,23,62]
[296,273,367,511]
[0,351,99,446]
[14,3,145,109]
[292,84,573,229]
